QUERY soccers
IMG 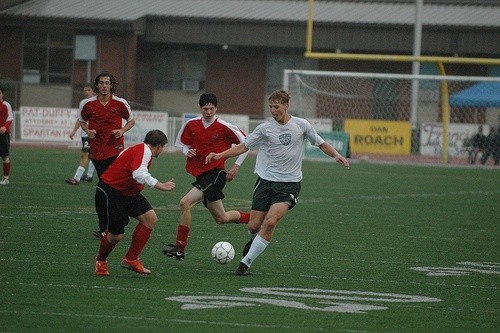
[210,240,235,265]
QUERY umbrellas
[449,81,500,108]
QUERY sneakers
[0,175,15,187]
[93,255,113,278]
[233,259,255,276]
[92,227,107,239]
[64,176,80,185]
[80,173,94,183]
[118,255,153,275]
[163,246,189,259]
[242,236,256,258]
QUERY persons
[468,125,500,164]
[0,85,14,184]
[64,83,97,185]
[94,129,176,276]
[78,70,135,239]
[204,89,350,274]
[161,92,251,261]
